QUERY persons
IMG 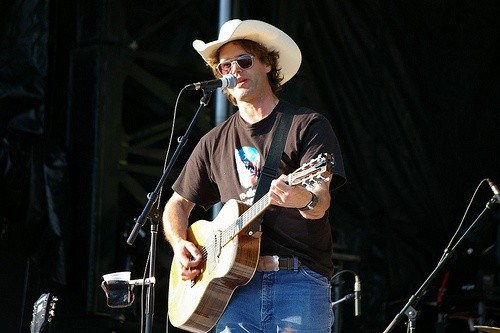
[161,18,347,333]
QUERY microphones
[184,73,238,90]
[487,178,500,202]
[355,274,362,316]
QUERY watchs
[299,191,319,212]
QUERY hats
[192,19,302,88]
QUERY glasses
[216,54,261,76]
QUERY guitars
[166,150,336,333]
[29,292,60,333]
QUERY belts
[257,255,306,272]
